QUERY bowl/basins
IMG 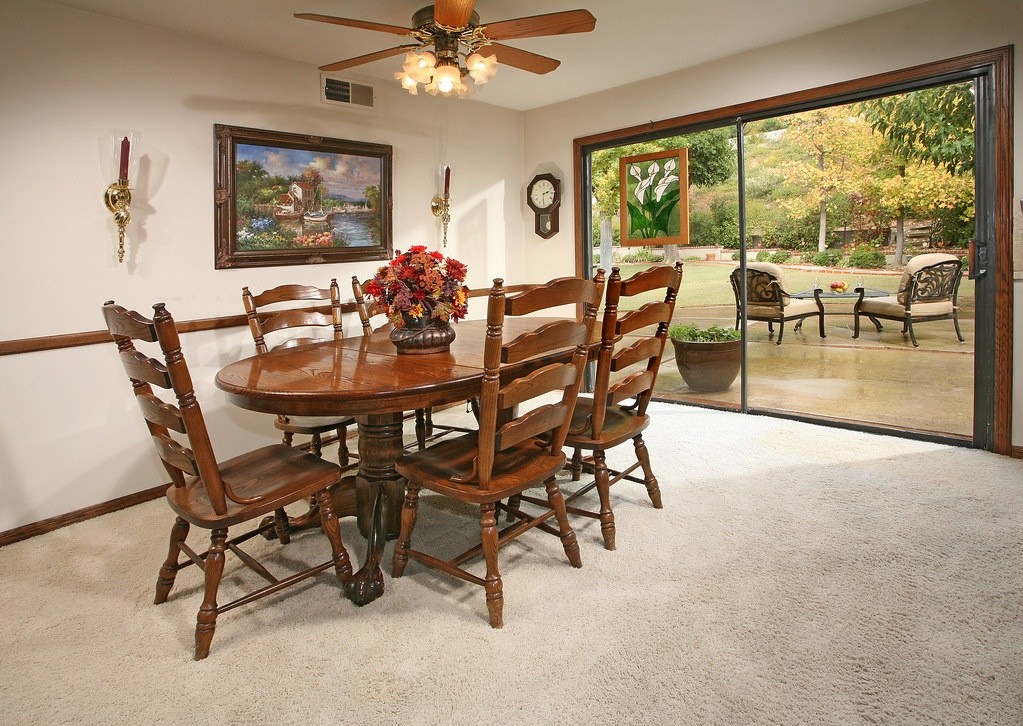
[825,282,851,295]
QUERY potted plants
[668,321,742,393]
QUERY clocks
[527,172,561,239]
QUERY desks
[214,317,624,610]
[788,291,890,332]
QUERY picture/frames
[620,148,690,248]
[214,122,394,271]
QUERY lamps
[393,50,497,99]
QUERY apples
[830,281,847,293]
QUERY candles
[444,166,451,194]
[119,136,131,180]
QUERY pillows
[734,261,791,307]
[897,253,959,306]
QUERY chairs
[730,268,828,345]
[385,268,606,628]
[101,298,356,664]
[242,277,358,509]
[351,273,480,456]
[506,262,684,551]
[851,260,966,347]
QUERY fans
[293,0,598,76]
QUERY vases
[388,309,457,354]
[706,253,716,261]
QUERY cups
[856,276,863,287]
[813,276,819,289]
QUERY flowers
[368,244,469,323]
